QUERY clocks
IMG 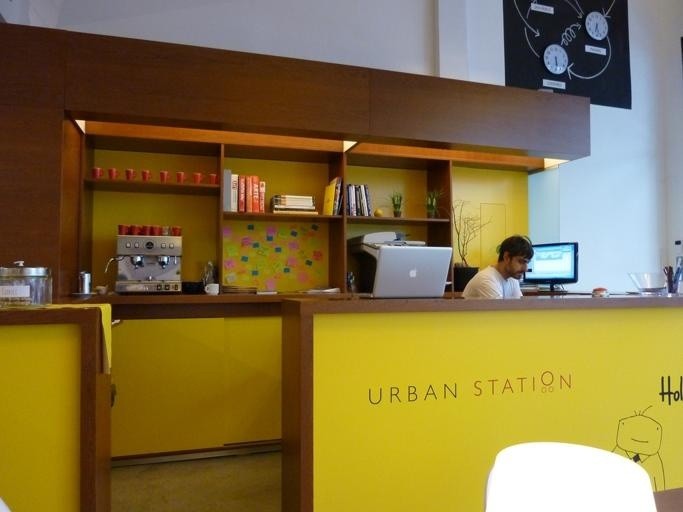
[544,44,568,75]
[586,12,609,40]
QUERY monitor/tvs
[523,242,579,292]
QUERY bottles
[78,269,94,295]
[0,266,53,310]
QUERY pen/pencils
[664,266,681,293]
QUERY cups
[663,274,681,295]
[204,283,220,296]
[90,165,218,186]
[114,222,184,237]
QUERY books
[223,167,372,217]
[519,279,564,292]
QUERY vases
[454,267,479,291]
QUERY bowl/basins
[625,271,670,295]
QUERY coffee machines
[104,234,182,294]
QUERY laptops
[355,246,452,298]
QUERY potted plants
[422,187,443,218]
[391,193,405,217]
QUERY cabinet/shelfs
[79,134,453,295]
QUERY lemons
[374,209,384,216]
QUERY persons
[463,235,535,300]
